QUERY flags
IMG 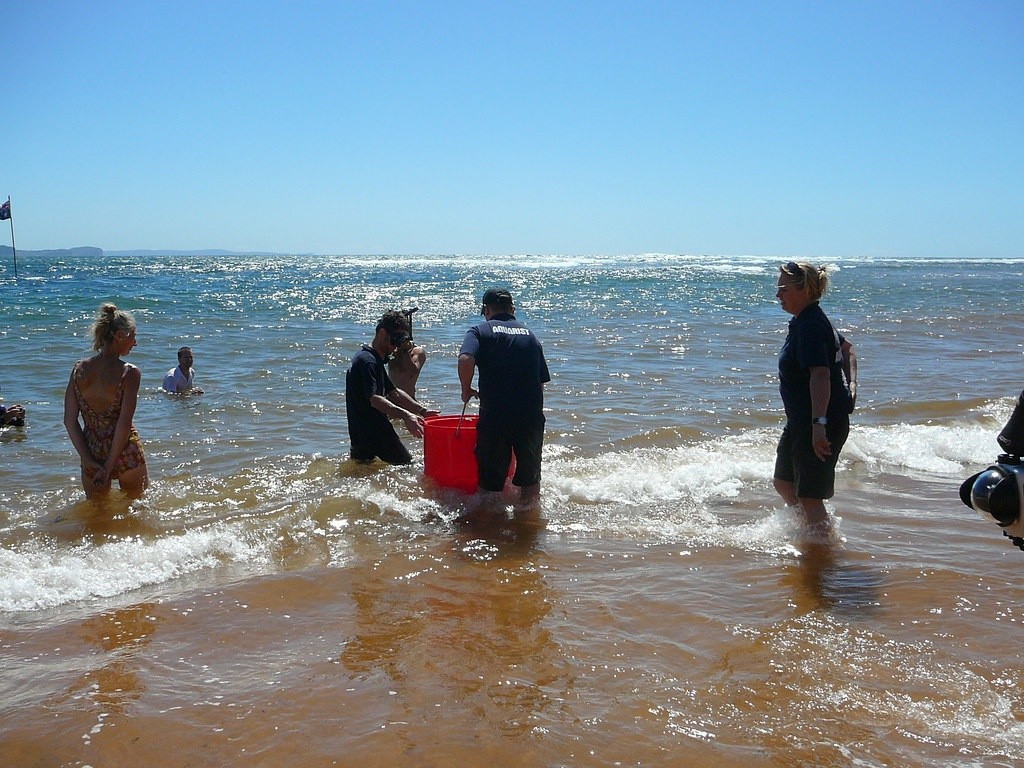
[0,200,10,220]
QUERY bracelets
[420,408,427,415]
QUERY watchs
[811,417,828,425]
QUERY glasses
[787,261,803,278]
[390,332,409,347]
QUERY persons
[458,288,550,493]
[164,347,197,395]
[346,309,441,465]
[0,387,25,427]
[63,302,149,497]
[773,261,857,533]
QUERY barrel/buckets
[421,392,514,494]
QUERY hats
[481,288,512,317]
